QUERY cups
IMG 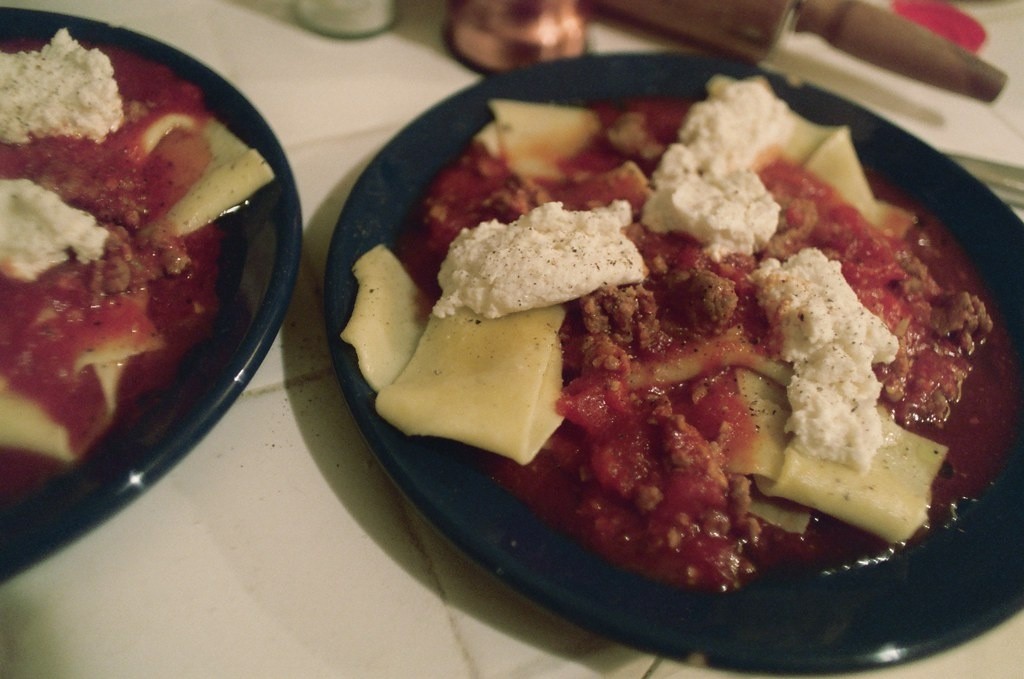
[296,0,398,35]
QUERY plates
[0,4,303,586]
[322,51,1024,678]
